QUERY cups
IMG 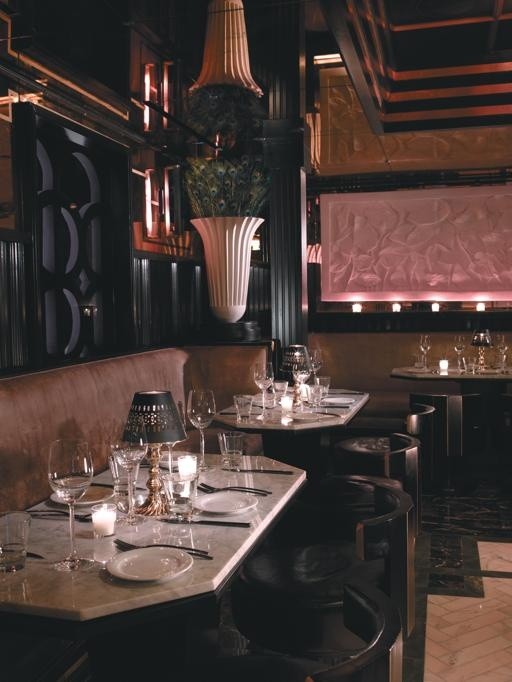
[0,509,32,573]
[231,347,332,423]
[217,430,245,469]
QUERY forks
[197,481,272,499]
[24,507,92,520]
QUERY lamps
[470,328,494,370]
[279,344,313,404]
[122,389,188,517]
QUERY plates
[107,549,191,583]
[194,488,259,518]
[322,396,356,406]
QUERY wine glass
[405,327,509,376]
[48,385,219,574]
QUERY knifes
[1,549,46,561]
[332,392,364,396]
[219,412,262,417]
[321,406,349,410]
[222,465,294,477]
[156,516,251,529]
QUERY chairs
[188,393,484,682]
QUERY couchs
[0,346,268,521]
